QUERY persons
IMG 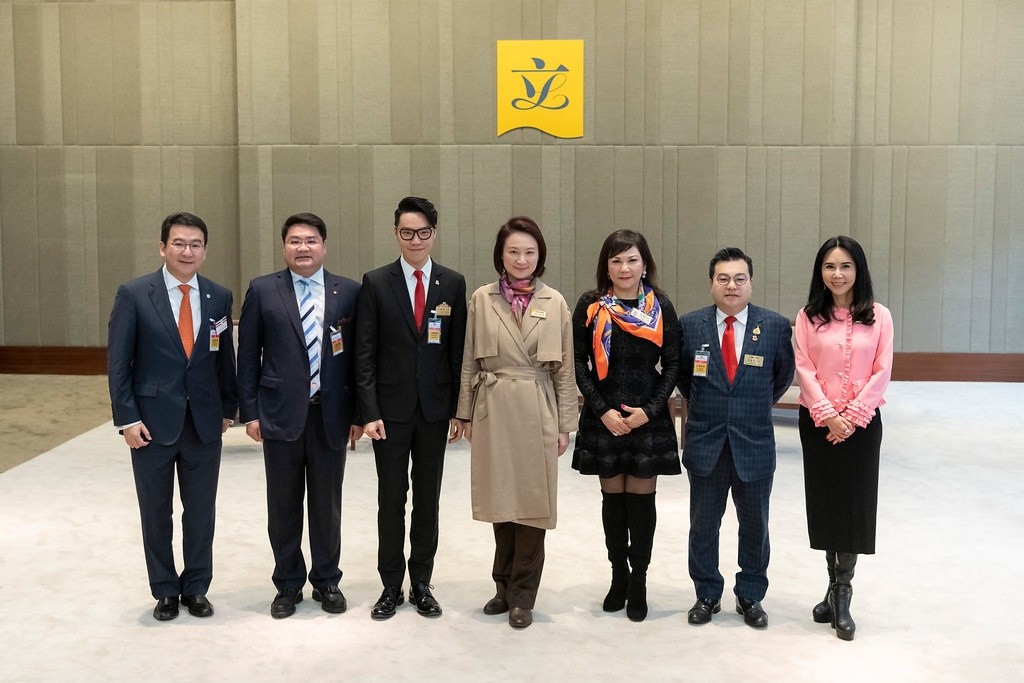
[355,197,467,619]
[795,236,894,641]
[237,213,364,619]
[106,215,239,619]
[677,248,796,626]
[456,214,580,628]
[572,229,681,622]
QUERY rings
[614,431,619,437]
[844,429,851,434]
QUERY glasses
[397,227,433,240]
[711,273,748,286]
[286,238,320,246]
[165,241,205,252]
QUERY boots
[603,560,630,611]
[626,558,648,621]
[830,561,856,640]
[813,563,835,624]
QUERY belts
[308,394,322,407]
[486,367,553,381]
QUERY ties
[721,316,738,385]
[177,285,194,360]
[413,269,425,335]
[299,277,321,398]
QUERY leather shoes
[270,588,303,618]
[408,584,442,616]
[180,593,214,617]
[370,585,404,618]
[312,587,347,613]
[688,598,721,624]
[483,596,509,615]
[509,606,533,627]
[154,595,180,621]
[735,595,768,627]
